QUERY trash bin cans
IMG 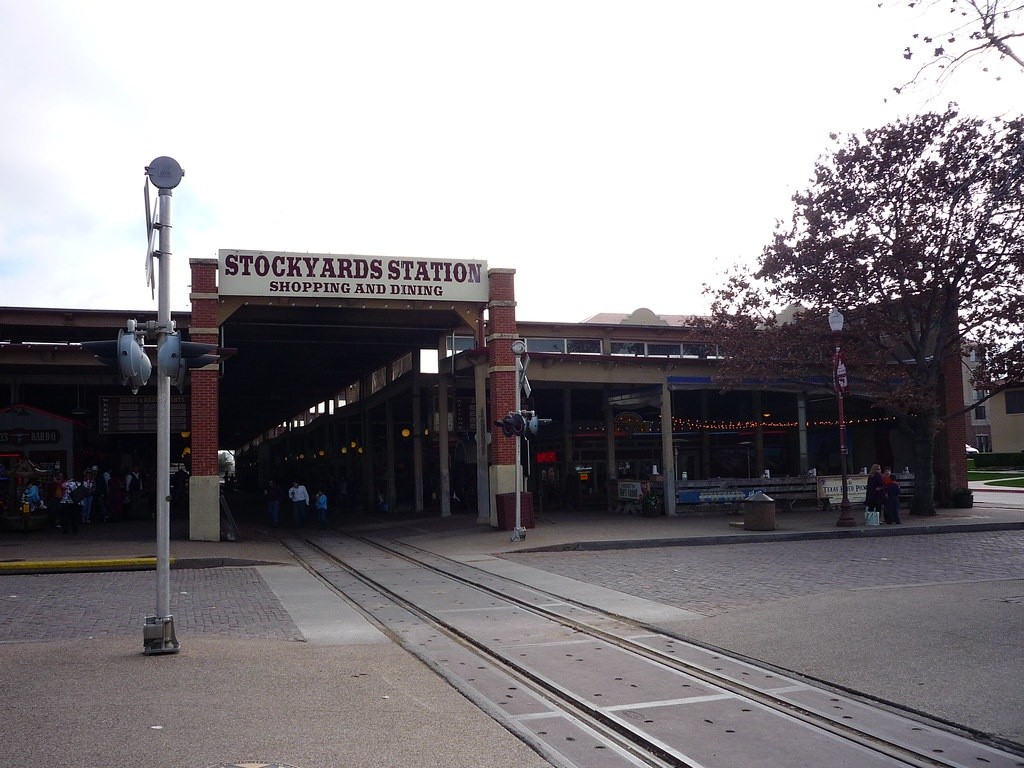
[742,493,776,530]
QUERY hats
[890,473,898,480]
[91,465,99,471]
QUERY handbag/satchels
[864,507,879,526]
[68,480,89,504]
[15,493,30,514]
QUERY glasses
[876,468,881,470]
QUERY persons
[47,462,113,534]
[327,473,361,512]
[20,480,41,517]
[102,462,156,522]
[289,480,311,530]
[863,463,904,525]
[316,489,328,529]
[264,469,285,531]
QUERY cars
[965,444,979,454]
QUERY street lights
[829,307,858,528]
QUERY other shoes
[880,521,883,524]
[896,522,903,525]
[81,520,86,524]
[886,521,892,524]
[87,519,94,525]
